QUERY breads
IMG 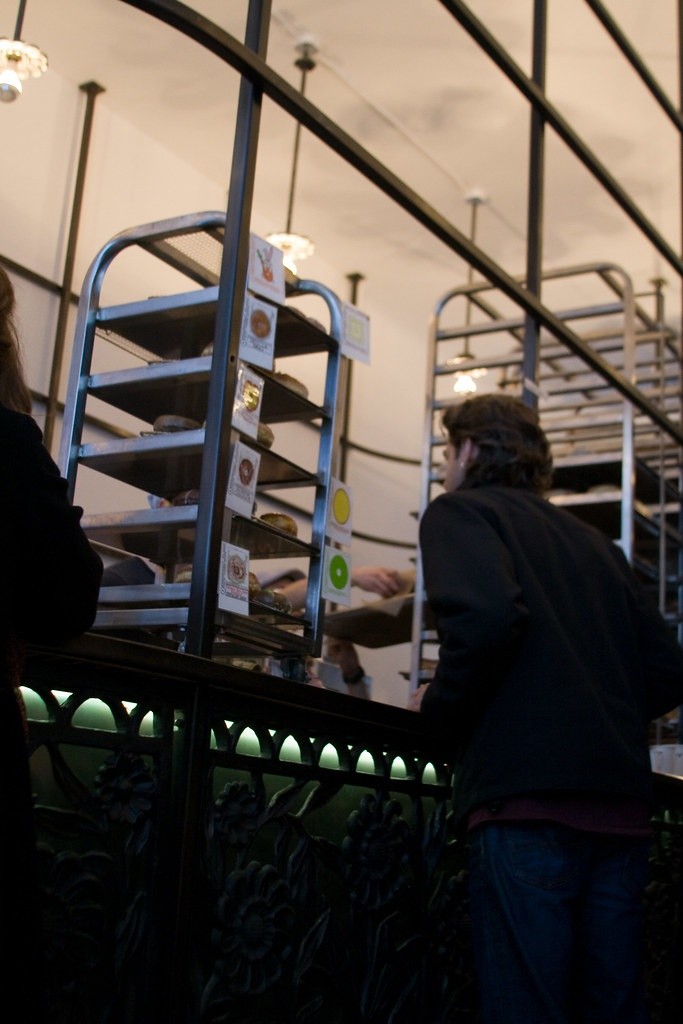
[148,306,328,617]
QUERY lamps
[0,0,49,102]
[445,188,488,395]
[264,31,320,275]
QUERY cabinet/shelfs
[58,210,346,684]
[405,256,683,703]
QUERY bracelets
[343,670,365,684]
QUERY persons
[0,267,105,1024]
[419,395,683,1024]
[94,493,195,646]
[216,566,399,700]
